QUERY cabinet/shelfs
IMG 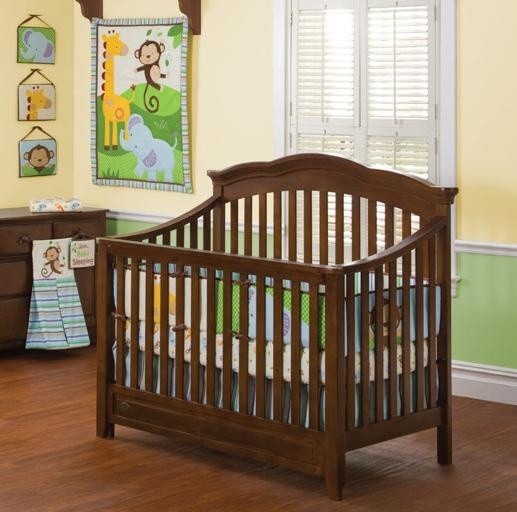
[0,205,108,355]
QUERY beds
[93,153,463,499]
